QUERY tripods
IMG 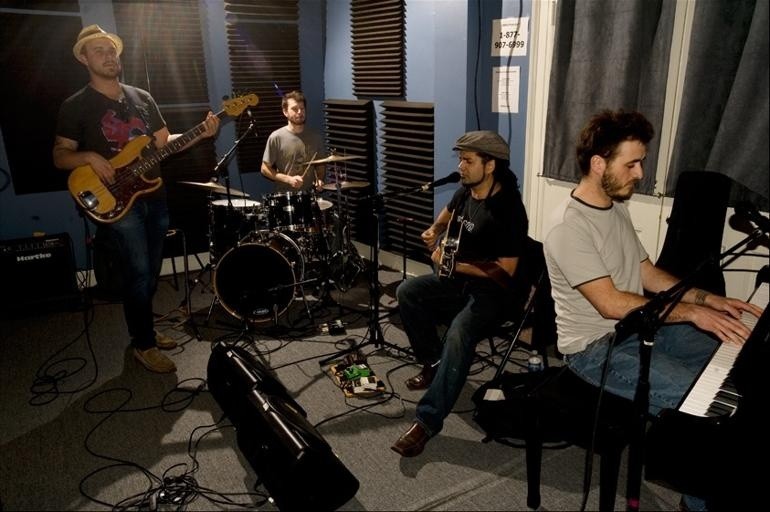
[318,186,419,366]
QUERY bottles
[528,350,541,372]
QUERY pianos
[644,264,770,510]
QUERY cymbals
[177,180,249,198]
[324,181,370,189]
[306,155,365,165]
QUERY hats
[73,24,123,64]
[451,130,509,161]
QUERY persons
[392,115,526,454]
[53,23,219,375]
[542,108,761,512]
[260,90,328,193]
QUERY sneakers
[132,347,175,374]
[154,329,175,348]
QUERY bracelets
[696,290,710,304]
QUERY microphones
[246,108,259,138]
[419,171,462,192]
[732,197,770,234]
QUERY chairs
[463,239,547,383]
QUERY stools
[470,361,641,510]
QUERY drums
[317,199,334,225]
[263,190,316,229]
[214,230,304,322]
[211,198,261,219]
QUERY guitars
[432,218,463,279]
[67,88,259,223]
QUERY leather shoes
[390,421,429,457]
[404,367,435,390]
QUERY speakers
[234,389,360,512]
[206,339,307,428]
